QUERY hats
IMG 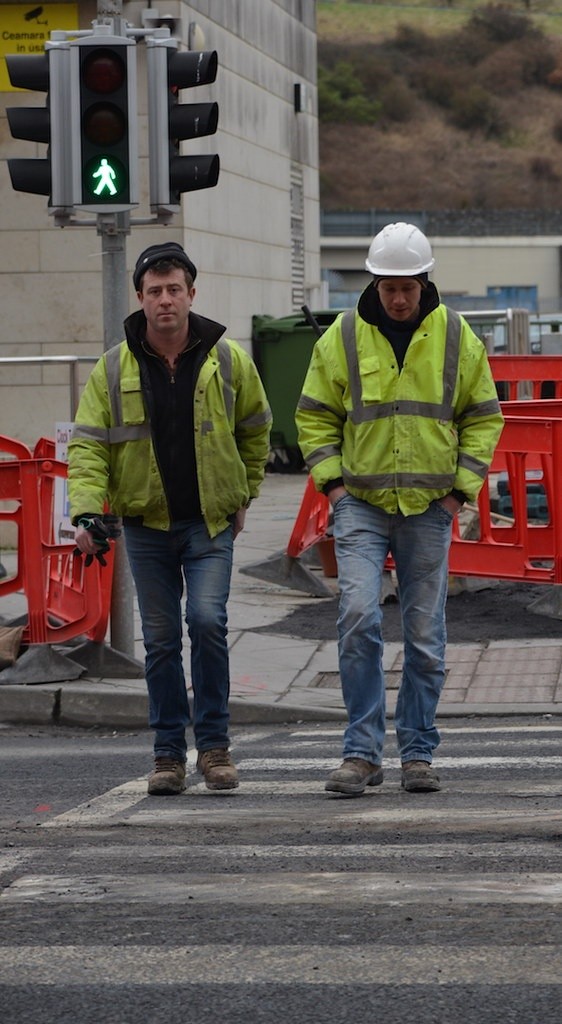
[132,241,197,289]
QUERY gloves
[74,517,111,568]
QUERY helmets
[365,223,436,275]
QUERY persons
[67,242,273,794]
[295,221,506,795]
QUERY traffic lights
[69,36,142,213]
[3,42,72,216]
[149,35,221,210]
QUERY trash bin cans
[250,312,338,474]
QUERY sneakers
[401,761,441,790]
[323,758,385,794]
[148,758,186,792]
[197,748,240,790]
